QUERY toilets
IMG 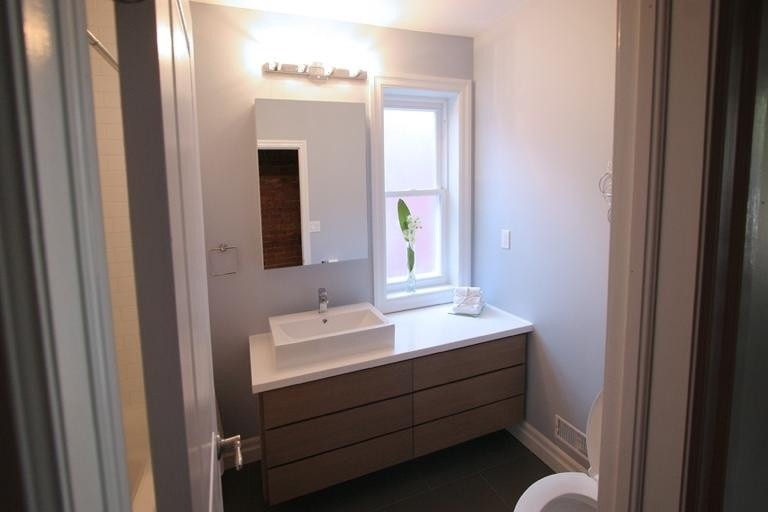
[513,386,602,512]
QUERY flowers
[395,194,425,272]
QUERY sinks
[268,302,395,371]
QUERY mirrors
[253,97,371,274]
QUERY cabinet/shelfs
[246,328,534,511]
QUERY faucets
[318,287,329,312]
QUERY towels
[447,285,487,317]
